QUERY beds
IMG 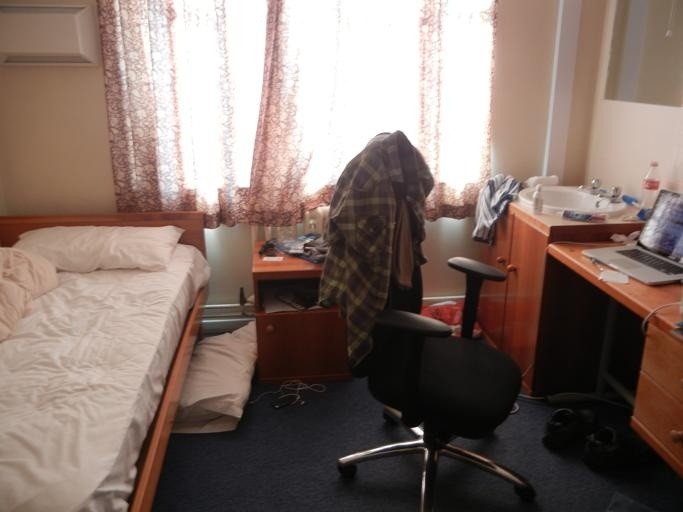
[1,211,210,512]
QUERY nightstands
[252,240,353,385]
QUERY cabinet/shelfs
[476,202,647,397]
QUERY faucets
[578,176,601,195]
[596,184,621,208]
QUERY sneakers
[542,409,622,474]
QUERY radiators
[251,204,330,240]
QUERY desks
[546,240,682,483]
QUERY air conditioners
[0,3,98,68]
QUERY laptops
[581,188,682,287]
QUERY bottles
[533,184,544,215]
[640,160,660,209]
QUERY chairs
[335,130,537,511]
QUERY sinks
[517,186,626,216]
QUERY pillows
[12,224,187,274]
[171,320,260,436]
